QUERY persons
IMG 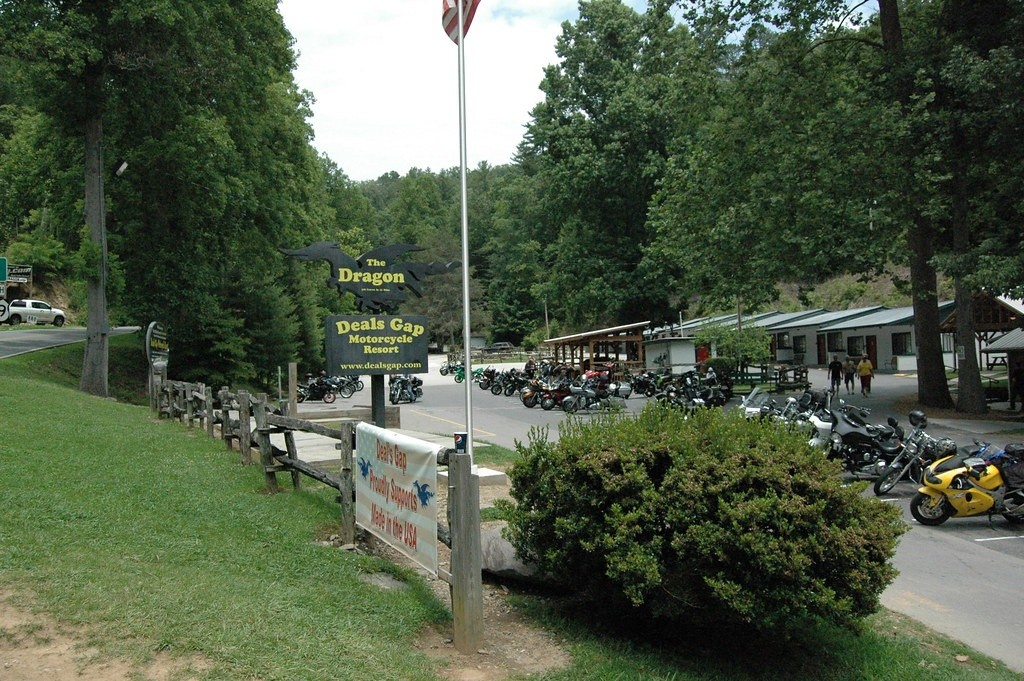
[526,356,537,371]
[659,353,667,367]
[857,354,874,397]
[388,375,415,400]
[630,349,635,367]
[828,355,843,392]
[842,355,857,395]
[716,370,730,389]
[707,367,717,383]
[605,361,615,371]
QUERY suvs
[5,299,66,327]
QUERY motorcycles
[909,435,1024,525]
[388,374,424,405]
[294,371,364,405]
[439,350,959,495]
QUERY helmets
[936,438,957,459]
[909,411,927,428]
[785,397,797,406]
